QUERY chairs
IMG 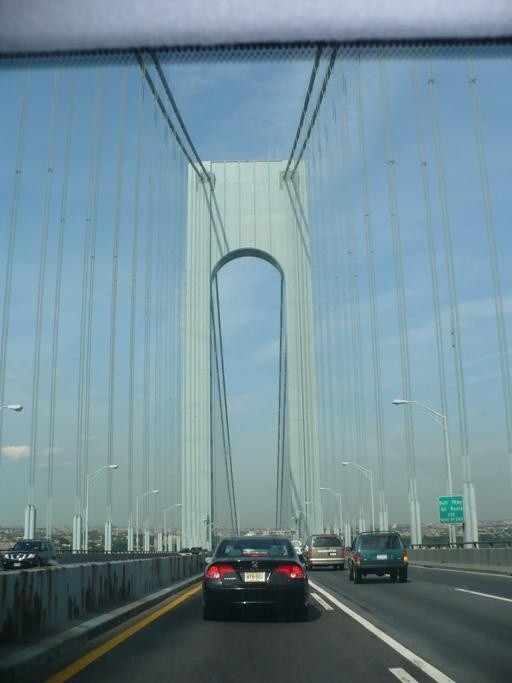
[228,548,243,557]
[268,544,284,557]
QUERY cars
[290,534,345,570]
[347,532,409,583]
[201,535,309,619]
[3,538,56,567]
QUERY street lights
[291,487,342,534]
[342,461,374,532]
[136,490,161,549]
[163,504,182,552]
[390,399,457,549]
[86,464,120,551]
